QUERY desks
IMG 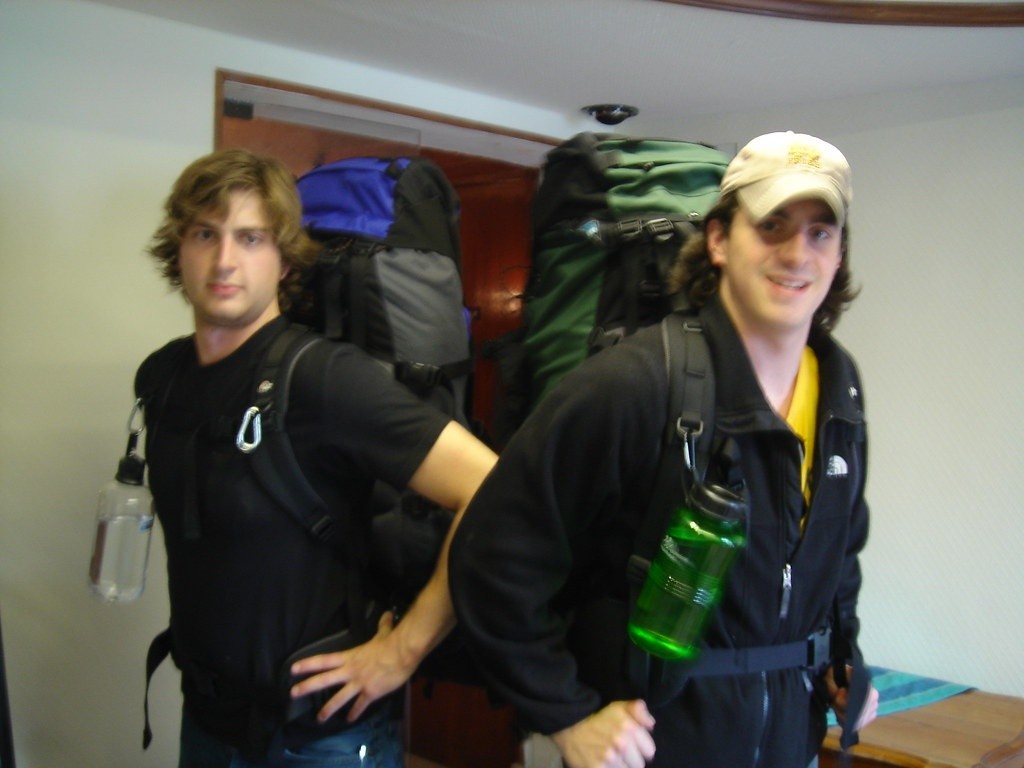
[816,664,1024,768]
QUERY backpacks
[139,150,481,754]
[494,132,838,695]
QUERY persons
[447,129,882,768]
[132,151,499,768]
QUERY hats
[716,131,852,230]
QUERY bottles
[88,452,153,604]
[625,478,748,663]
